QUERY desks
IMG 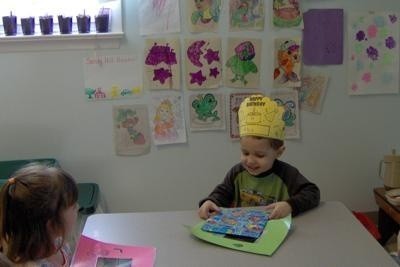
[68,200,399,266]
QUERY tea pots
[378,148,400,191]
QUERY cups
[3,15,109,36]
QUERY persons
[198,135,320,219]
[0,162,80,267]
